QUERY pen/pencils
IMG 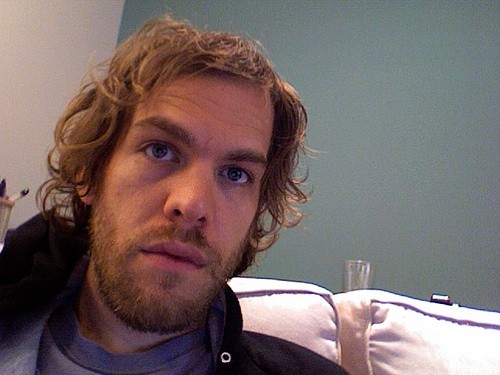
[0,177,30,205]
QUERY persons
[0,15,351,375]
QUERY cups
[0,198,15,244]
[342,259,374,293]
